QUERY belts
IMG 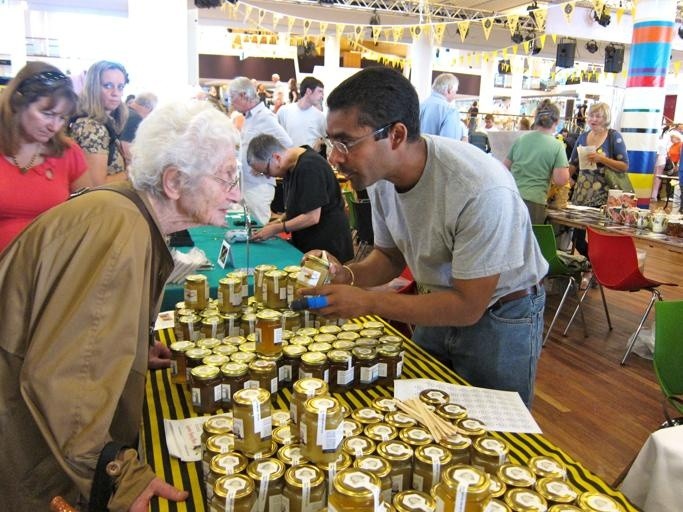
[491,279,545,312]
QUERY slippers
[580,276,591,290]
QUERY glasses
[320,125,395,157]
[198,171,241,195]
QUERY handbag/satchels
[604,165,635,198]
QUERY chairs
[563,225,679,365]
[532,224,589,347]
[345,192,374,261]
[655,301,682,414]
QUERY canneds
[203,378,618,511]
[169,254,404,416]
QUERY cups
[600,189,683,238]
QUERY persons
[417,72,628,257]
[74,60,129,191]
[652,121,683,217]
[225,73,356,265]
[121,94,158,162]
[293,63,552,410]
[0,93,244,512]
[2,59,90,257]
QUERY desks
[656,174,680,209]
[138,311,639,512]
[548,206,683,262]
[161,209,304,309]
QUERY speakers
[604,47,624,72]
[557,42,575,67]
